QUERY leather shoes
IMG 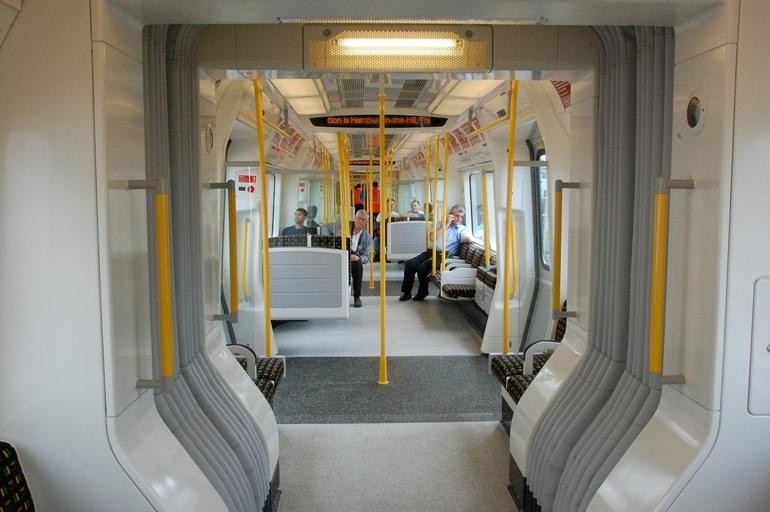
[413,292,429,301]
[400,293,412,301]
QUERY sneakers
[354,298,361,307]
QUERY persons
[320,210,370,307]
[374,199,400,249]
[399,205,472,301]
[421,203,433,220]
[307,205,319,228]
[372,182,380,217]
[282,208,309,235]
[354,184,364,210]
[410,200,420,212]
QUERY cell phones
[450,211,454,214]
